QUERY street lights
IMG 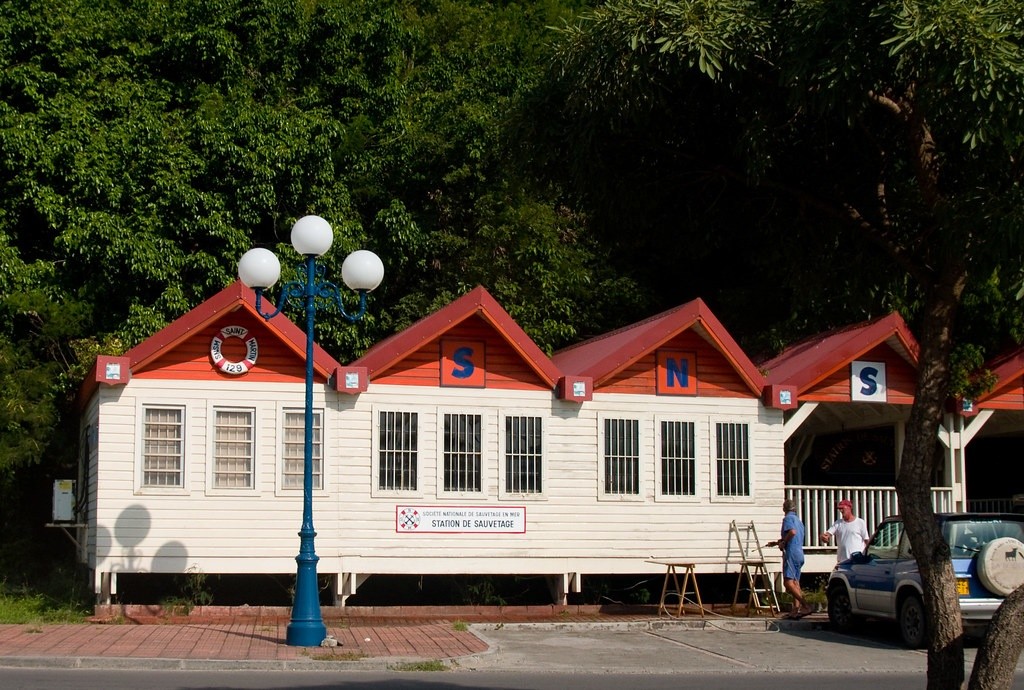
[238,215,385,647]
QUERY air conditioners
[52,479,77,522]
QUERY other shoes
[785,608,798,617]
[799,607,811,615]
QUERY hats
[838,500,852,508]
[783,499,797,510]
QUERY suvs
[827,512,1023,649]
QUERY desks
[644,559,781,619]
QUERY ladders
[731,519,781,615]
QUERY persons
[821,500,870,564]
[767,500,812,620]
